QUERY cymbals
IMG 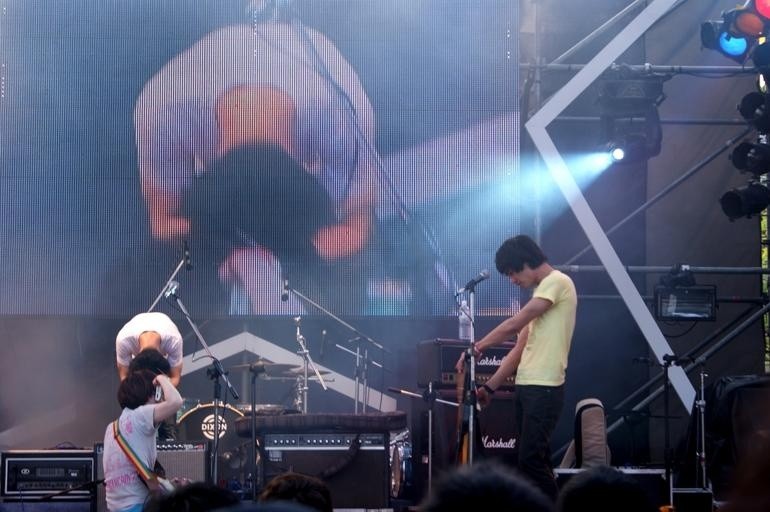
[277,367,329,379]
[227,362,299,372]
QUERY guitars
[145,466,176,498]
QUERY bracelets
[475,340,484,354]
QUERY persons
[142,455,673,512]
[115,311,183,441]
[131,22,379,315]
[103,368,184,512]
[99,236,309,314]
[454,234,578,503]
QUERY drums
[391,440,414,500]
[229,404,282,419]
[175,400,261,490]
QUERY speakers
[398,388,521,501]
[260,431,389,508]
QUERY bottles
[458,300,472,340]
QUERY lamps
[598,73,667,161]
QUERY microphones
[183,240,192,271]
[280,276,290,301]
[455,269,490,297]
